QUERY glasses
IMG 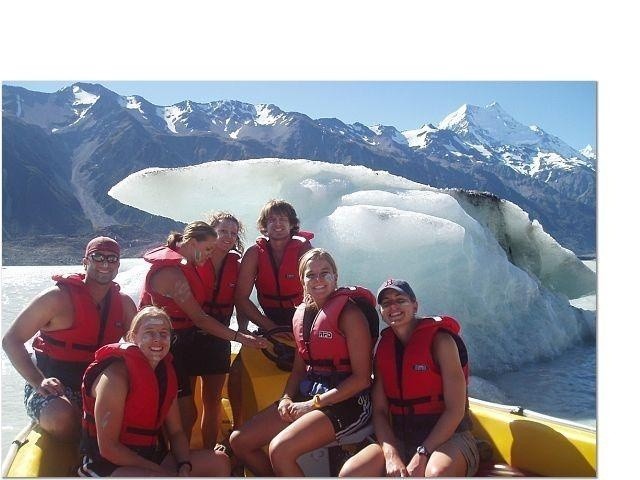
[91,252,119,263]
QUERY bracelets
[280,393,297,401]
[177,460,192,472]
[313,394,320,407]
[233,331,237,341]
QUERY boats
[0,353,597,477]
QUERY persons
[77,306,231,477]
[138,221,269,450]
[229,248,379,477]
[2,236,138,442]
[190,212,253,450]
[339,280,479,477]
[234,198,314,341]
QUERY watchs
[417,445,431,458]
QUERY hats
[376,278,417,306]
[85,236,121,256]
